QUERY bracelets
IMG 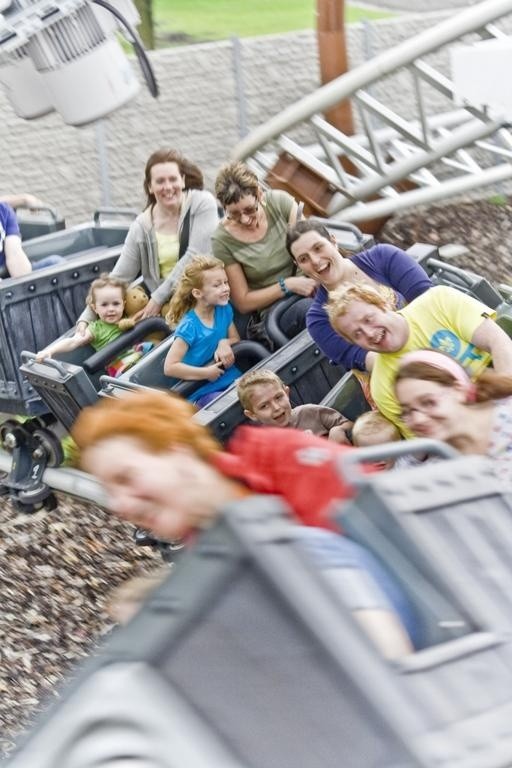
[276,275,292,299]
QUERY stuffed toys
[118,285,170,348]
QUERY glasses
[224,195,258,221]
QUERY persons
[323,279,512,433]
[284,219,434,375]
[237,369,354,449]
[0,199,66,282]
[393,347,512,479]
[33,271,155,380]
[350,410,428,475]
[210,161,316,342]
[164,255,242,412]
[71,144,218,346]
[69,392,423,664]
